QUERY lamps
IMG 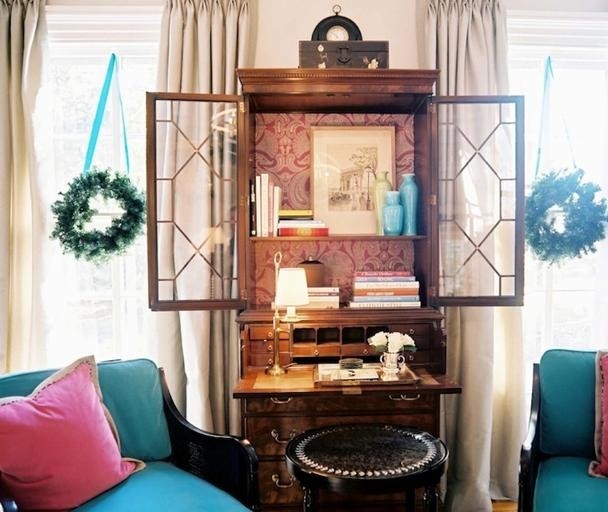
[518,348,608,511]
[1,358,259,512]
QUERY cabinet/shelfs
[147,68,525,512]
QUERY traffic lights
[1,356,145,510]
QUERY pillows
[1,356,145,510]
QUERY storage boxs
[300,40,388,68]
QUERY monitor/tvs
[250,172,330,236]
[271,269,422,309]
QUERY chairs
[1,358,259,512]
[518,348,608,511]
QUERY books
[300,40,388,68]
